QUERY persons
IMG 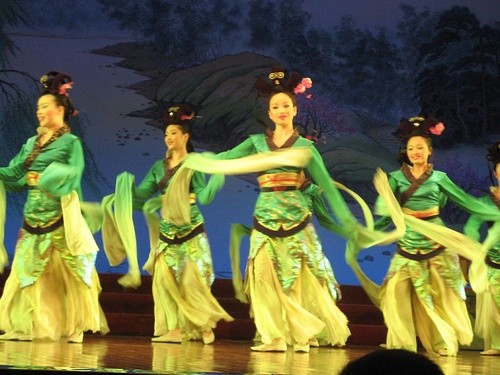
[0,71,110,343]
[182,70,352,353]
[114,104,235,344]
[463,143,500,355]
[374,116,500,357]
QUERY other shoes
[68,331,84,342]
[380,342,388,347]
[150,334,182,342]
[438,348,448,355]
[250,340,286,351]
[293,341,310,352]
[0,327,33,341]
[479,348,500,355]
[201,329,215,344]
[310,339,319,346]
